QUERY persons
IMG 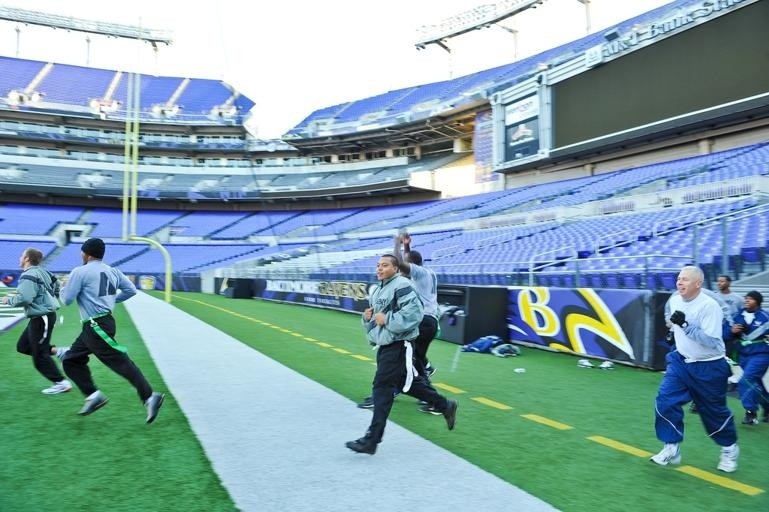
[345,253,458,455]
[2,248,72,396]
[647,264,742,473]
[57,237,166,424]
[357,232,444,417]
[721,289,769,427]
[714,274,745,319]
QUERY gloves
[670,311,688,328]
[665,331,675,346]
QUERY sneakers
[346,436,376,454]
[648,442,681,466]
[144,392,165,424]
[358,367,457,430]
[57,346,70,363]
[717,444,740,473]
[742,409,769,424]
[598,361,615,369]
[78,390,110,416]
[41,380,72,395]
[688,403,697,413]
[576,359,595,368]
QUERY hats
[81,238,105,259]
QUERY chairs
[537,206,769,291]
